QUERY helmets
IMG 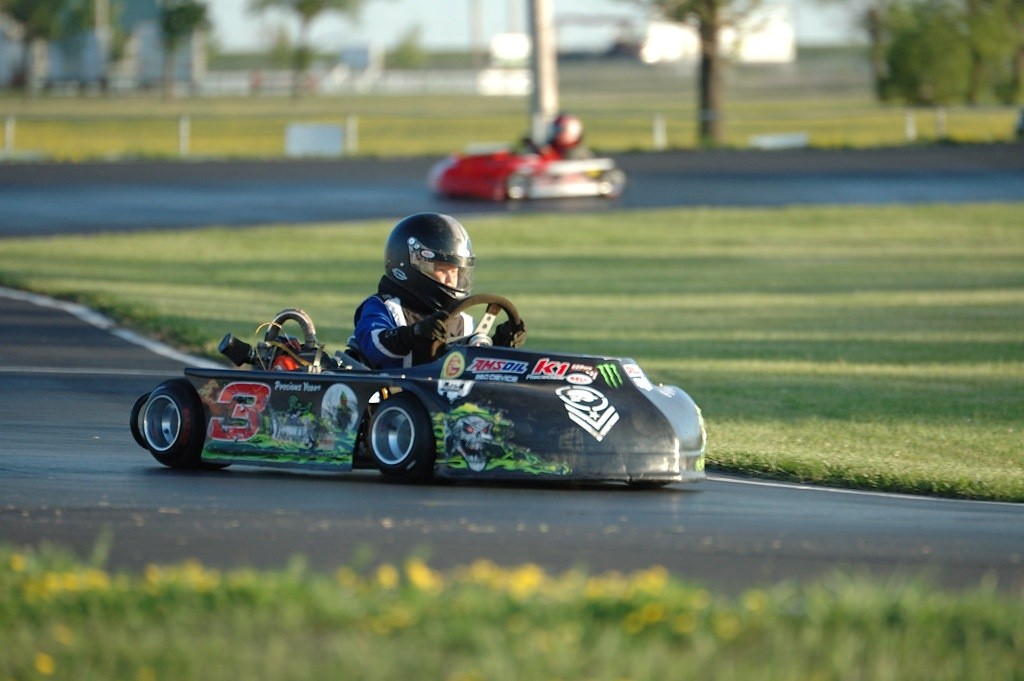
[384,210,475,313]
[554,114,583,144]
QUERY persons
[517,109,598,165]
[326,211,526,377]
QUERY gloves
[413,311,450,345]
[493,319,527,350]
